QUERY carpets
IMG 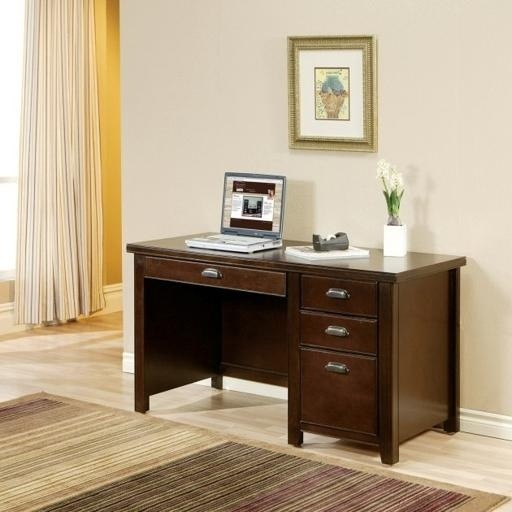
[0,392,512,512]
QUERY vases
[383,225,408,257]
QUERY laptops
[186,172,286,253]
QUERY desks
[125,233,467,467]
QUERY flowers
[376,159,407,225]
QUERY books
[286,246,368,262]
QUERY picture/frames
[287,35,380,151]
